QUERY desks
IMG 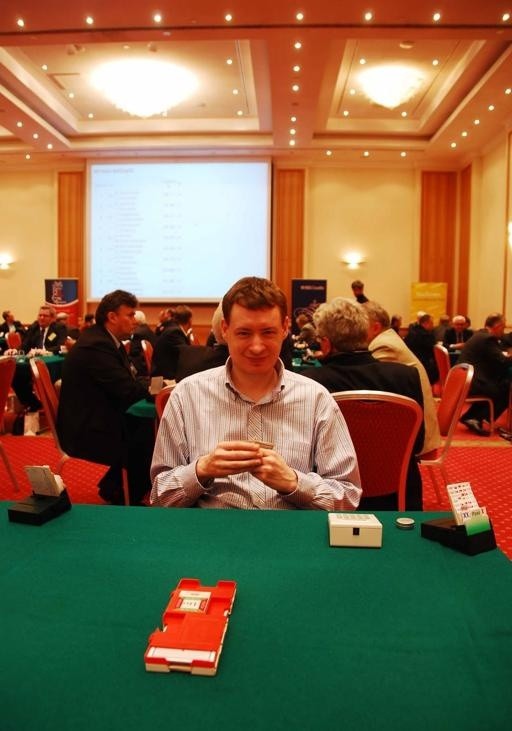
[10,355,65,406]
[0,505,512,730]
[123,400,161,466]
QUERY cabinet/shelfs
[411,283,448,329]
[45,278,79,332]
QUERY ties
[39,329,45,350]
[117,339,132,371]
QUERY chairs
[141,339,153,373]
[0,356,20,494]
[30,358,130,505]
[419,362,474,504]
[156,384,176,434]
[331,390,422,511]
[433,344,494,434]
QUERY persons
[57,312,79,340]
[403,314,440,385]
[57,290,156,506]
[298,299,427,511]
[1,311,27,348]
[351,280,368,305]
[443,316,472,352]
[166,309,174,326]
[177,298,293,384]
[129,311,156,378]
[152,305,193,388]
[11,305,67,411]
[149,277,362,510]
[293,314,316,349]
[390,314,403,334]
[410,311,425,333]
[79,314,94,334]
[156,312,168,341]
[359,300,439,460]
[456,313,512,436]
[432,315,455,343]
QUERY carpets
[423,421,512,557]
[0,433,111,506]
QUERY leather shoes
[459,413,492,437]
